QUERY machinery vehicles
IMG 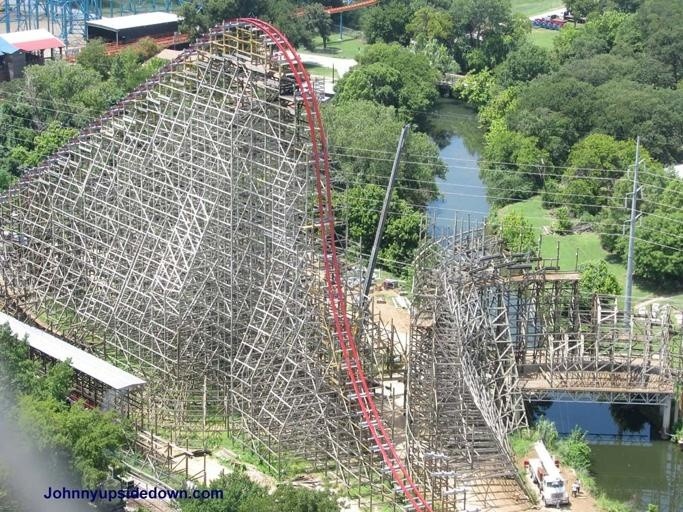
[528,441,569,507]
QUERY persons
[574,477,580,492]
[571,482,576,498]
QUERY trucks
[534,9,587,30]
